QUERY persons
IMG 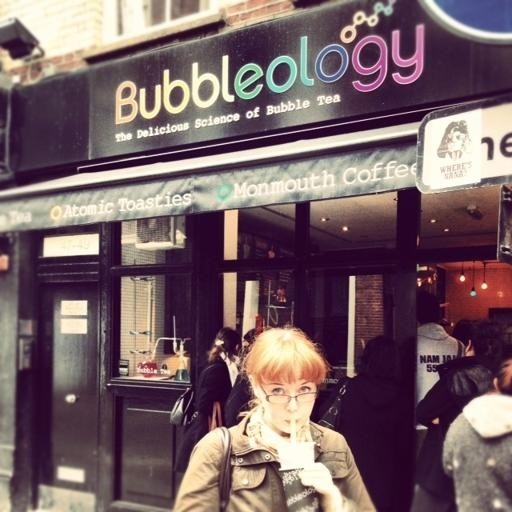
[319,333,412,512]
[175,328,259,474]
[174,328,375,512]
[411,292,512,510]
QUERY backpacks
[168,383,199,429]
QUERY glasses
[255,380,322,405]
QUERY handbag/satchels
[317,379,355,435]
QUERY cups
[279,468,318,512]
